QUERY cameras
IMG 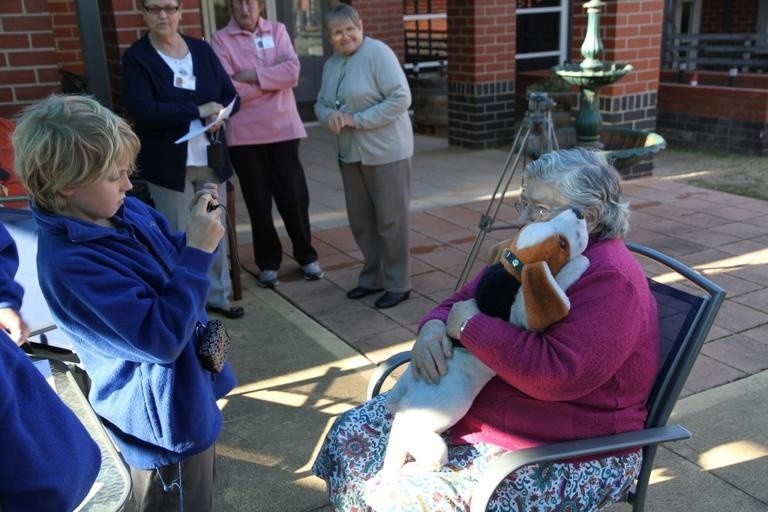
[189,189,216,213]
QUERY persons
[314,4,416,307]
[121,0,245,321]
[210,0,323,290]
[308,147,662,511]
[10,96,227,512]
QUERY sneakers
[301,259,324,281]
[257,270,280,288]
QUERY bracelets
[459,311,479,340]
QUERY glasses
[142,2,181,15]
[513,199,569,224]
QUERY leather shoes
[205,303,246,320]
[346,285,385,300]
[374,291,411,309]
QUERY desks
[25,354,137,512]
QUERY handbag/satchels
[204,125,234,184]
[195,318,232,383]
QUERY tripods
[451,92,561,294]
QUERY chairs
[318,239,727,510]
